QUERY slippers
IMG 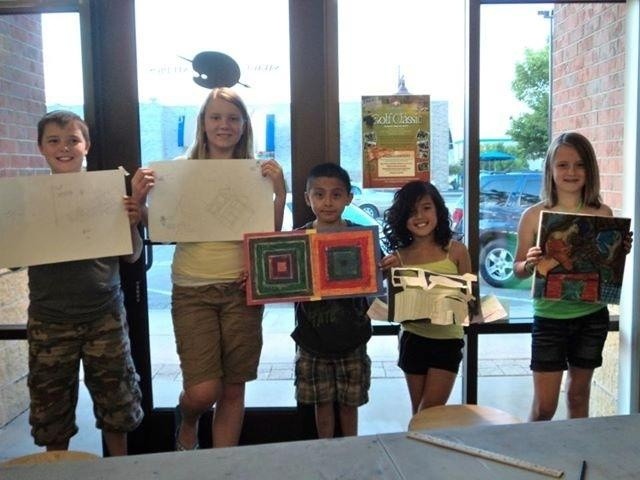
[174,406,200,452]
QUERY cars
[273,182,399,221]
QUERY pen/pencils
[579,460,586,480]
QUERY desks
[0,412,639,479]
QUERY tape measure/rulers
[407,431,563,478]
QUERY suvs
[449,170,544,288]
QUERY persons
[513,133,633,422]
[235,163,400,438]
[382,180,471,414]
[8,111,143,457]
[131,86,287,451]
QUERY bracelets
[524,261,533,275]
